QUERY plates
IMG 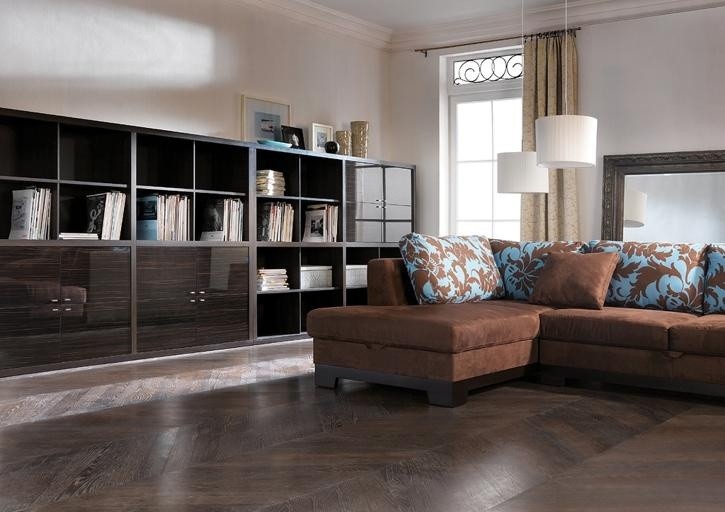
[256,139,292,148]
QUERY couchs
[305,257,724,408]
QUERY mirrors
[600,148,724,245]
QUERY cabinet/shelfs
[0,108,132,378]
[255,146,345,338]
[133,126,253,353]
[344,158,415,308]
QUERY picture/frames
[280,124,306,150]
[309,122,333,153]
[241,94,291,143]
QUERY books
[199,198,243,242]
[136,193,191,241]
[257,269,291,292]
[257,201,294,244]
[301,203,338,243]
[59,191,127,241]
[7,186,52,241]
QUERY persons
[290,133,302,150]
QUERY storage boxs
[345,265,368,286]
[301,265,333,290]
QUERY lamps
[535,0,598,170]
[496,0,550,194]
[624,190,647,228]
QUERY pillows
[586,237,711,316]
[702,242,724,315]
[487,238,592,302]
[527,249,620,310]
[399,232,507,306]
